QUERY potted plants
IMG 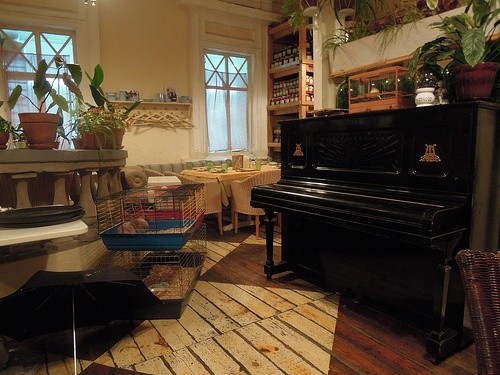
[280,0,500,98]
[0,54,128,149]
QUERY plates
[143,98,160,102]
[0,204,87,229]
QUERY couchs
[126,159,232,188]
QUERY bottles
[119,89,127,101]
[166,88,178,102]
[159,92,165,103]
[180,96,190,103]
[274,129,281,143]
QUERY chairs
[454,249,500,375]
[144,168,283,240]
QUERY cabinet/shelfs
[266,14,314,159]
[348,66,417,113]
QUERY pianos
[250,98,500,364]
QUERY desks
[0,204,88,248]
[182,163,280,231]
